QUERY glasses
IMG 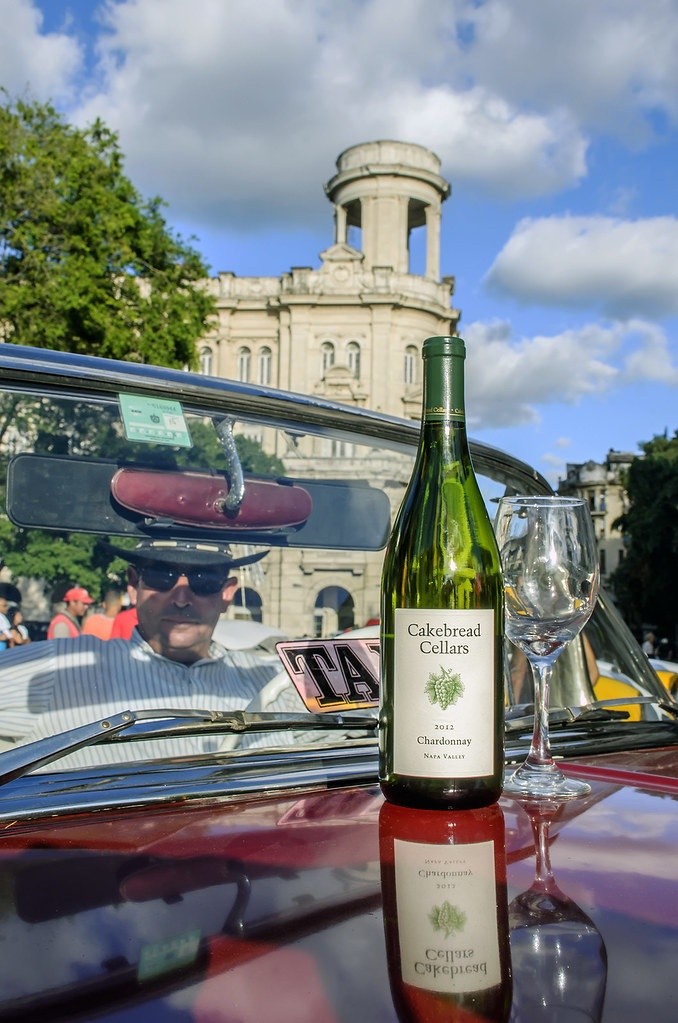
[133,563,228,598]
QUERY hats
[96,538,273,568]
[63,588,95,604]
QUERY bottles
[376,795,516,1023]
[376,332,507,808]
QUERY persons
[0,598,30,652]
[655,638,671,660]
[0,541,380,776]
[509,630,598,709]
[47,587,96,641]
[110,607,138,640]
[642,633,655,659]
[82,580,123,641]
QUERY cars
[0,341,677,1022]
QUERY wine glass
[504,798,609,1022]
[489,493,594,799]
